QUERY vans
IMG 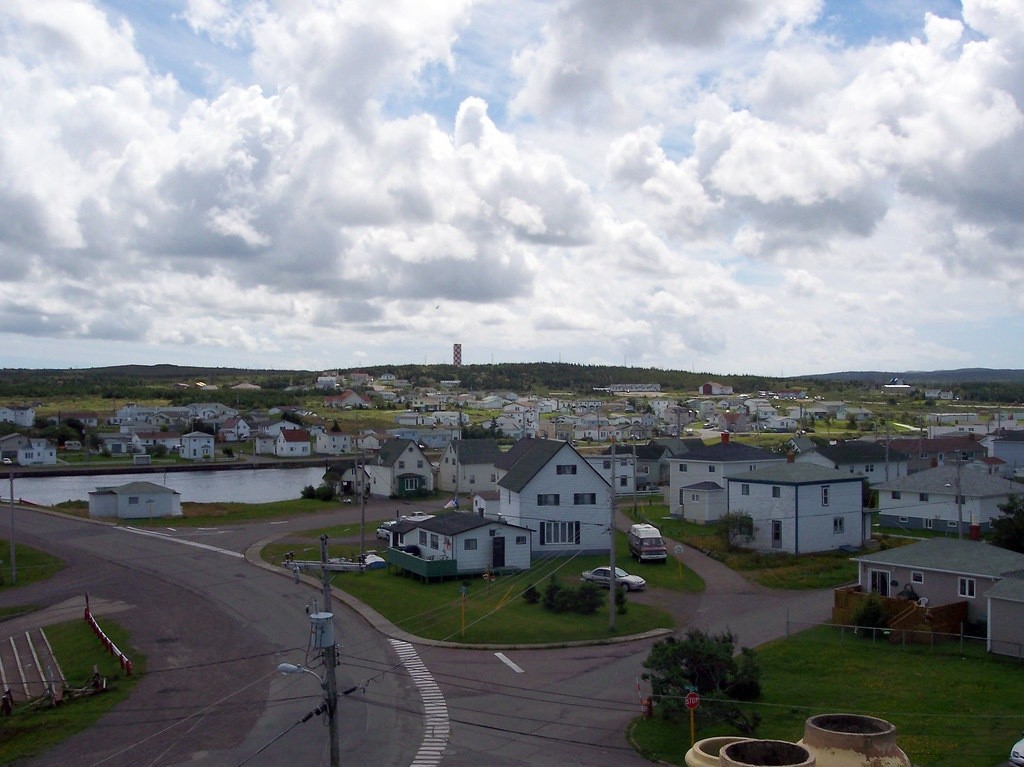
[703,423,712,429]
[629,522,668,563]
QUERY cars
[172,383,188,389]
[581,566,646,592]
[3,457,12,465]
[1009,738,1024,767]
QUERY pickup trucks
[401,511,435,522]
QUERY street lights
[944,483,963,541]
[278,662,340,767]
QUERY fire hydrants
[644,697,655,719]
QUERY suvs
[377,520,396,540]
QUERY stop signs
[685,692,701,710]
